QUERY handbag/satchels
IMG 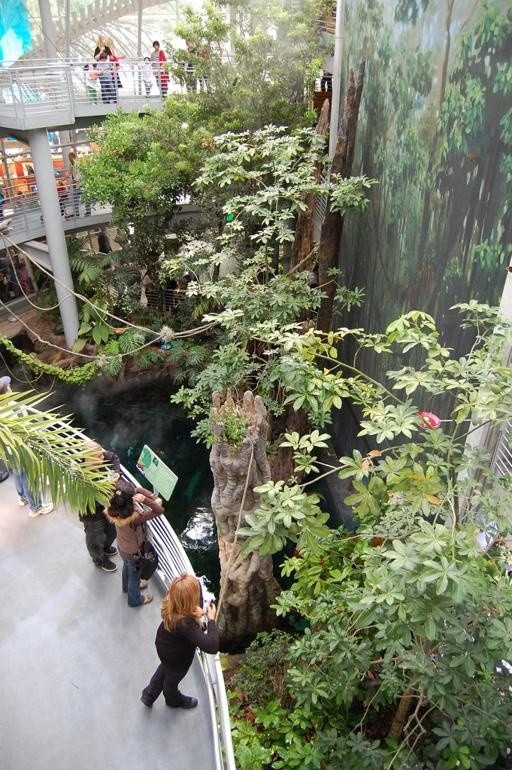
[141,540,158,580]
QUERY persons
[57,180,67,203]
[140,573,219,710]
[143,265,196,311]
[62,151,90,217]
[83,34,213,104]
[0,375,56,517]
[0,184,6,221]
[103,488,164,607]
[99,226,113,269]
[0,249,50,306]
[79,441,135,572]
[321,70,333,92]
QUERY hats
[0,375,11,393]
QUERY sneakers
[29,503,53,517]
[19,496,28,506]
[166,696,198,709]
[140,691,152,707]
[140,579,148,588]
[143,594,152,603]
[104,545,117,556]
[101,559,116,572]
